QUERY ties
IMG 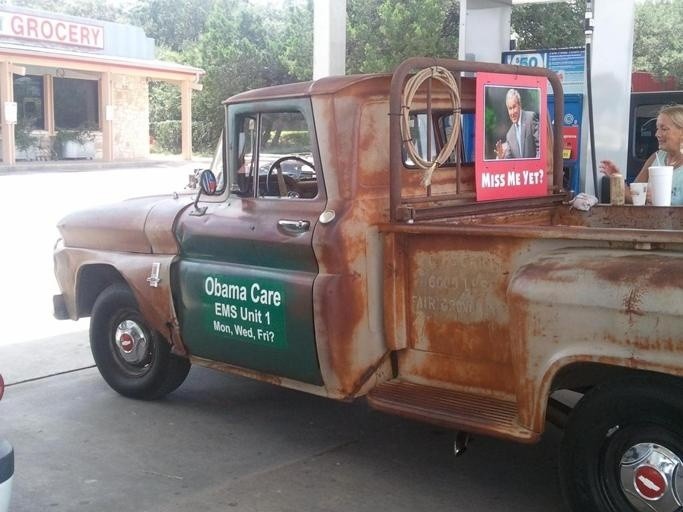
[515,123,521,151]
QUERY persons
[494,89,539,157]
[598,105,683,206]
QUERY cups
[647,165,673,207]
[629,182,648,206]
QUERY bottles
[609,173,624,204]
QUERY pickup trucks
[53,56,683,511]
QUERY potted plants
[56,120,96,159]
